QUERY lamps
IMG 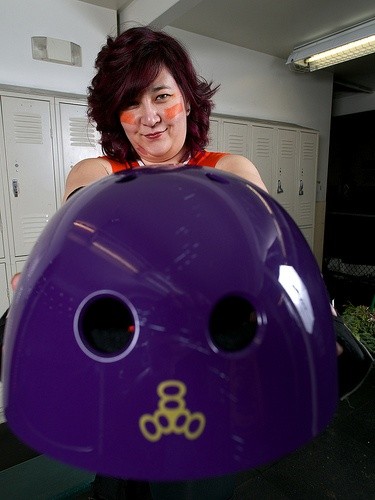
[285,18,375,73]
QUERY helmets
[2,163,341,482]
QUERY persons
[10,25,343,500]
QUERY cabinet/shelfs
[0,83,320,319]
